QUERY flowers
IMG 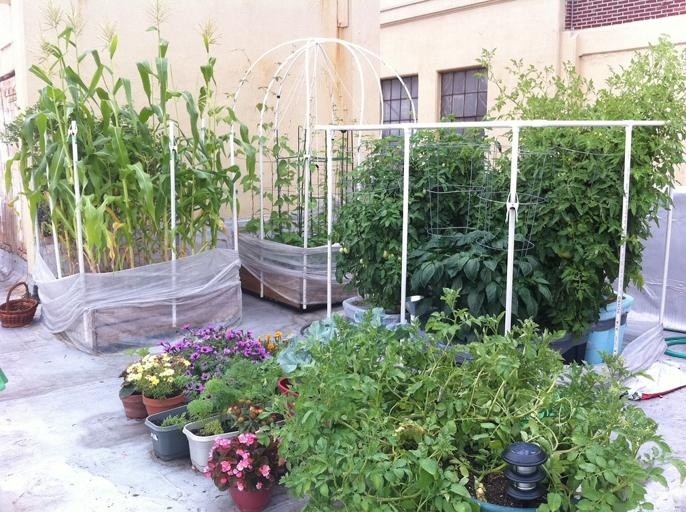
[121,352,190,398]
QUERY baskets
[0,281,38,328]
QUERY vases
[139,390,188,419]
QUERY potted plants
[144,285,685,512]
[118,350,147,420]
